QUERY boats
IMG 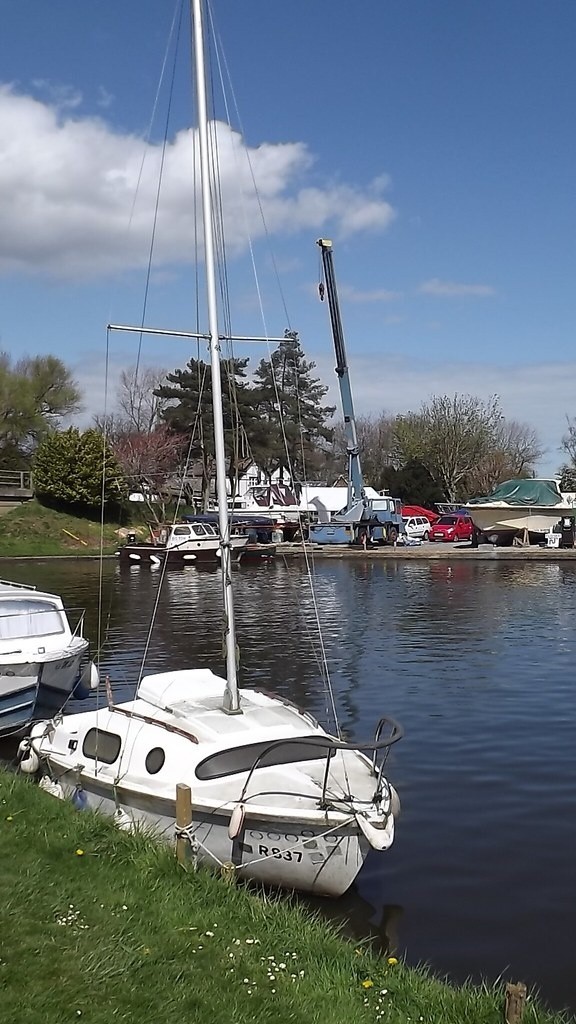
[115,522,250,563]
[0,574,102,747]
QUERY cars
[429,514,474,542]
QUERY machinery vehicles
[315,239,403,547]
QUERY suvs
[403,516,431,540]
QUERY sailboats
[15,0,406,903]
